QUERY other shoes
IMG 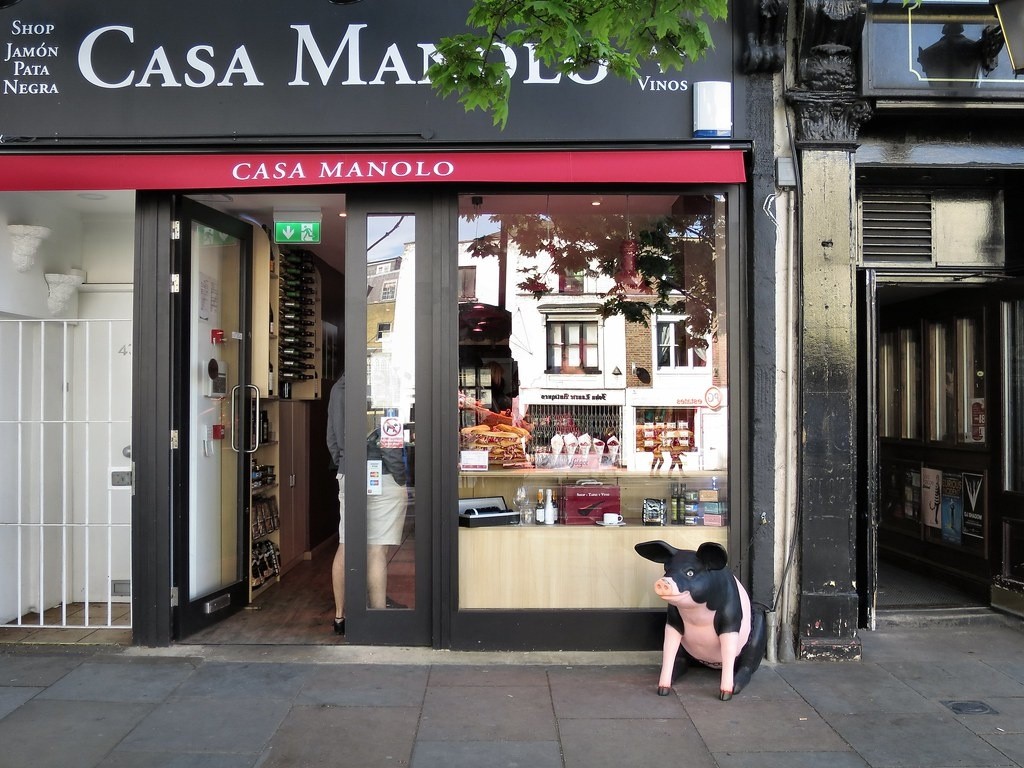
[333,620,346,635]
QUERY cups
[604,513,623,525]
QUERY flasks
[465,506,500,515]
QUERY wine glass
[512,485,530,527]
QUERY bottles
[534,492,545,525]
[277,249,315,383]
[678,483,687,525]
[268,351,273,396]
[270,246,274,271]
[671,482,679,525]
[252,410,268,444]
[270,303,274,334]
[544,489,555,525]
[551,489,560,524]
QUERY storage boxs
[458,496,520,528]
[558,480,621,525]
[700,490,726,526]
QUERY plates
[596,521,626,527]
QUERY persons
[560,338,619,452]
[650,427,689,480]
[326,320,408,636]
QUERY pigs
[634,540,766,701]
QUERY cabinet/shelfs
[220,222,323,603]
[458,468,729,609]
